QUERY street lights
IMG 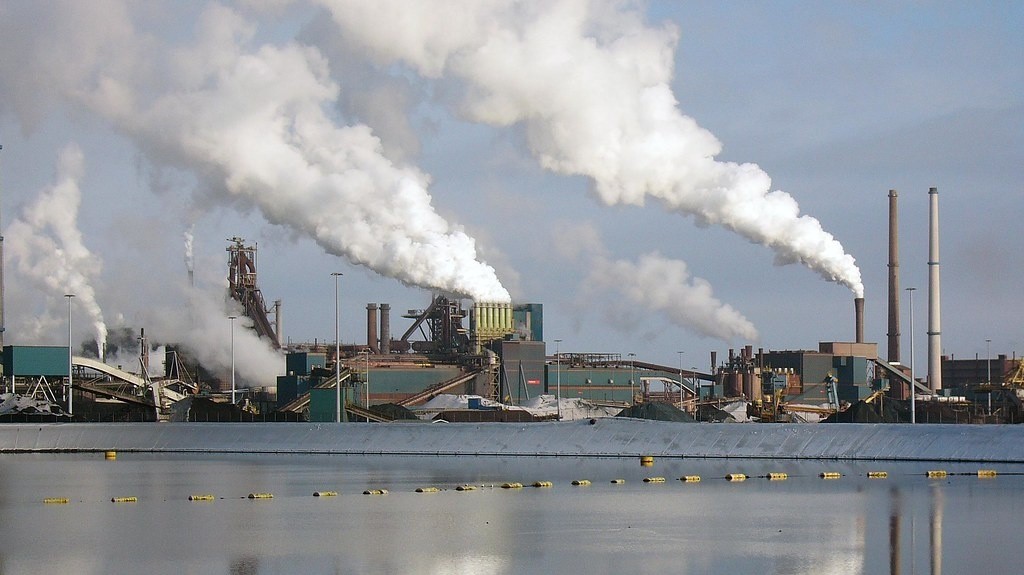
[906,288,917,423]
[627,353,637,406]
[678,351,684,411]
[64,294,75,414]
[228,316,237,405]
[555,339,562,421]
[691,366,697,421]
[985,339,992,416]
[331,273,343,422]
